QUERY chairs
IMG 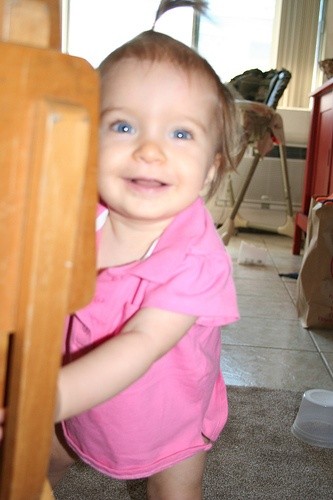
[216,68,295,246]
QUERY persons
[0,0,242,500]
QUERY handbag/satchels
[294,194,333,331]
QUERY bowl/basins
[291,389,333,449]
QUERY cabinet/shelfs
[292,77,333,256]
